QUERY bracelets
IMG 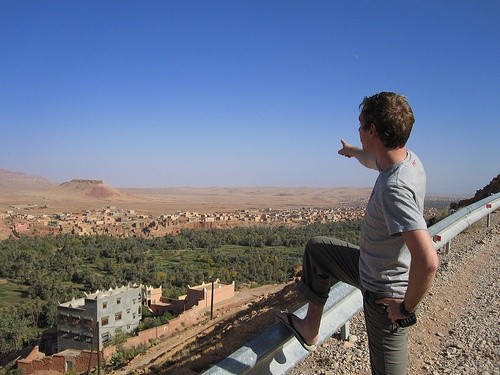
[399,300,416,318]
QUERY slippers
[275,311,318,351]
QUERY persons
[274,91,439,375]
